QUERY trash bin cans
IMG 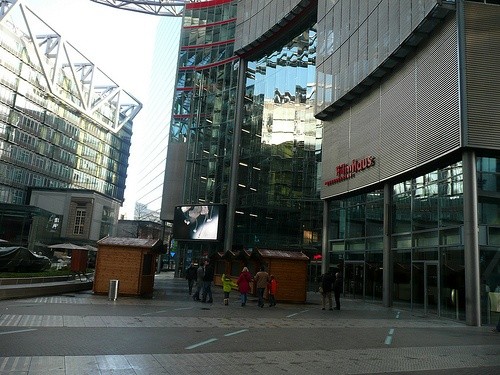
[108,279,120,301]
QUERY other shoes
[321,308,326,310]
[329,308,333,310]
[334,308,339,310]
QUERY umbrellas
[49,242,99,253]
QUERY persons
[185,260,276,309]
[332,273,341,309]
[320,269,333,310]
[33,251,96,267]
